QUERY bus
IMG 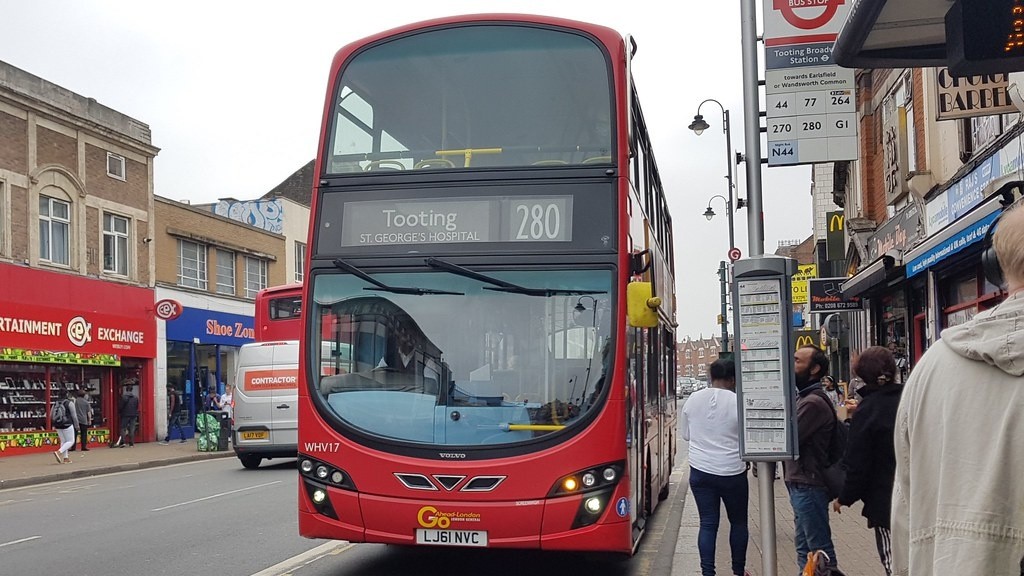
[254,284,386,377]
[300,13,680,556]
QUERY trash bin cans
[206,411,228,451]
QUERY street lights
[192,337,211,452]
[685,98,736,361]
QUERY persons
[848,376,867,403]
[824,375,844,403]
[159,383,188,446]
[681,358,750,576]
[376,324,439,390]
[49,388,80,463]
[204,386,221,410]
[888,198,1024,575]
[780,344,846,576]
[68,389,92,452]
[118,385,138,448]
[214,383,232,442]
[831,345,904,576]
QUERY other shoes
[81,448,90,451]
[752,461,758,478]
[775,467,781,480]
[63,458,73,464]
[130,443,134,447]
[159,440,168,446]
[54,451,62,463]
[180,440,187,443]
[119,443,126,448]
[68,447,75,451]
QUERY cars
[675,378,709,400]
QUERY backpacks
[812,389,852,499]
[51,399,68,424]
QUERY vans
[233,341,388,471]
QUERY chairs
[361,158,456,171]
[458,364,584,402]
[533,155,610,165]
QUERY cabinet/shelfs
[0,388,96,432]
